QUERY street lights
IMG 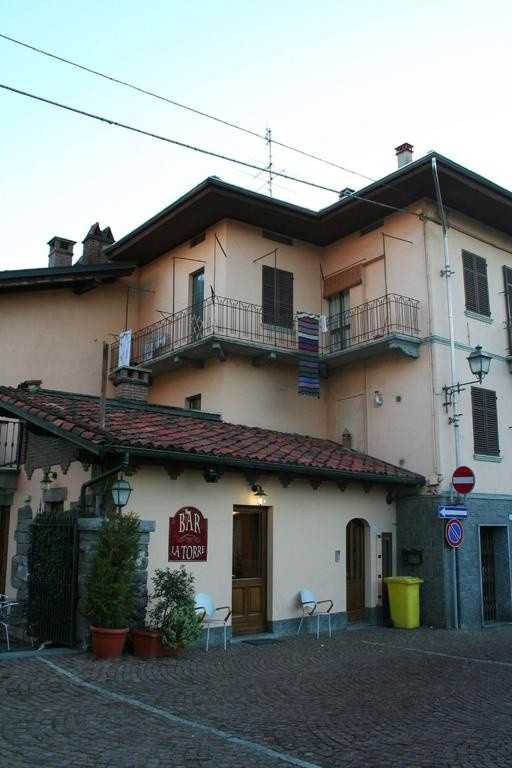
[112,472,135,518]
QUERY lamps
[112,475,134,513]
[40,470,59,490]
[443,345,492,413]
[251,482,267,504]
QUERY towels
[320,316,328,333]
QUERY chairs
[195,594,231,652]
[1,594,53,651]
[296,591,334,643]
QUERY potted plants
[134,568,203,660]
[84,509,142,659]
[160,600,206,657]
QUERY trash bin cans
[383,576,425,629]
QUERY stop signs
[451,464,476,495]
